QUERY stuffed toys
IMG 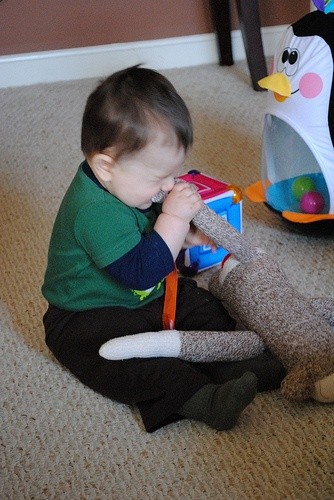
[97,178,334,403]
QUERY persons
[41,64,289,434]
